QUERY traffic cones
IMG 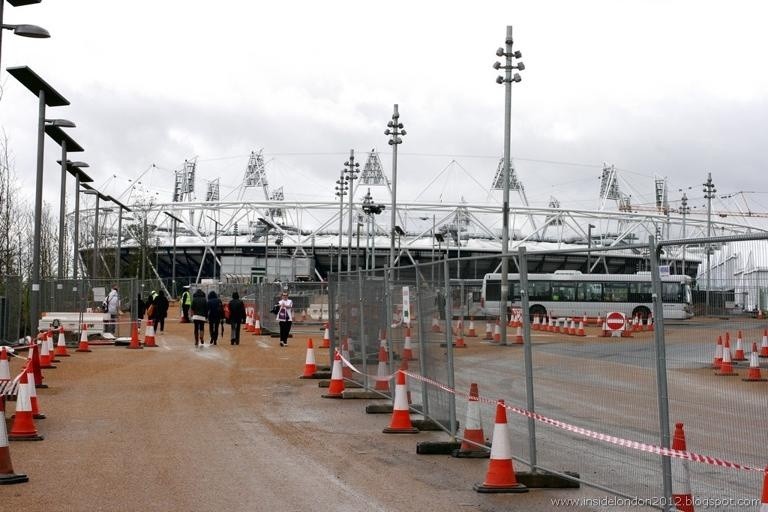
[300,323,421,434]
[473,399,530,494]
[0,325,71,484]
[761,465,767,512]
[712,329,767,382]
[300,309,308,321]
[448,384,490,457]
[672,422,695,511]
[428,310,611,347]
[622,312,653,337]
[76,323,93,352]
[126,319,158,350]
[292,310,296,321]
[758,309,763,319]
[243,309,264,336]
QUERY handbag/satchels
[102,303,108,312]
[272,303,289,322]
[147,305,155,316]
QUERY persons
[151,290,169,335]
[276,292,293,347]
[181,285,192,323]
[137,293,145,320]
[102,285,121,332]
[146,296,154,320]
[190,289,208,346]
[434,289,446,321]
[228,292,246,345]
[207,291,225,345]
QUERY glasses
[282,294,287,296]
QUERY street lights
[492,24,524,346]
[335,147,383,279]
[385,102,406,282]
[703,171,715,317]
[676,186,696,276]
[420,214,438,294]
[587,224,597,273]
[206,214,285,282]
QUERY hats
[151,291,156,294]
[183,286,190,288]
[435,289,440,293]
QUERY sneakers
[138,329,141,334]
[195,336,217,345]
[279,340,288,346]
[231,338,239,345]
[160,332,164,334]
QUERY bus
[448,278,496,317]
[481,267,696,326]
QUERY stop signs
[606,312,625,330]
